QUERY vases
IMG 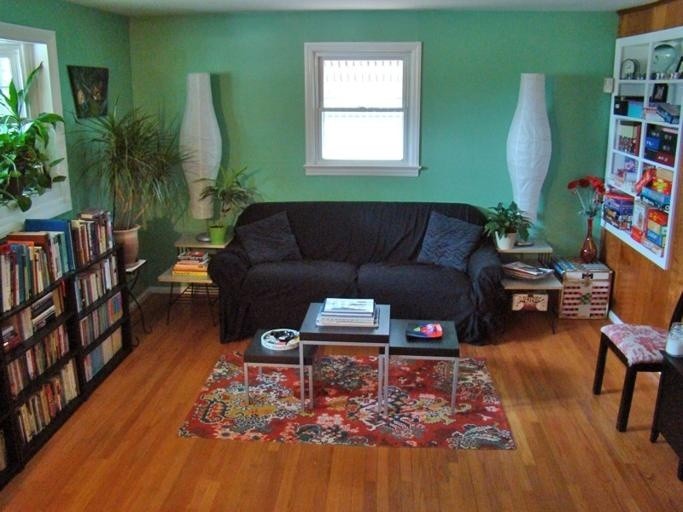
[578,215,600,264]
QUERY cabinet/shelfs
[0,207,128,491]
[494,237,562,335]
[649,291,682,481]
[601,24,683,270]
[157,232,233,327]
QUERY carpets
[176,346,517,452]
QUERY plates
[621,58,638,80]
[405,321,443,339]
[651,43,678,76]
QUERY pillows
[234,208,302,265]
[416,211,484,274]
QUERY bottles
[664,322,683,358]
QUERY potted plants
[483,199,531,250]
[1,58,63,210]
[65,98,187,263]
[194,166,259,245]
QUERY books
[500,258,555,279]
[0,207,127,470]
[169,250,212,282]
[317,297,379,328]
[613,119,640,156]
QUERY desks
[378,318,460,415]
[297,299,390,419]
[241,328,316,407]
[119,259,147,348]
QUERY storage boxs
[550,255,614,322]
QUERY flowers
[565,174,608,217]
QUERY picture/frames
[66,64,109,119]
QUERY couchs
[208,200,502,341]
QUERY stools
[591,324,671,432]
